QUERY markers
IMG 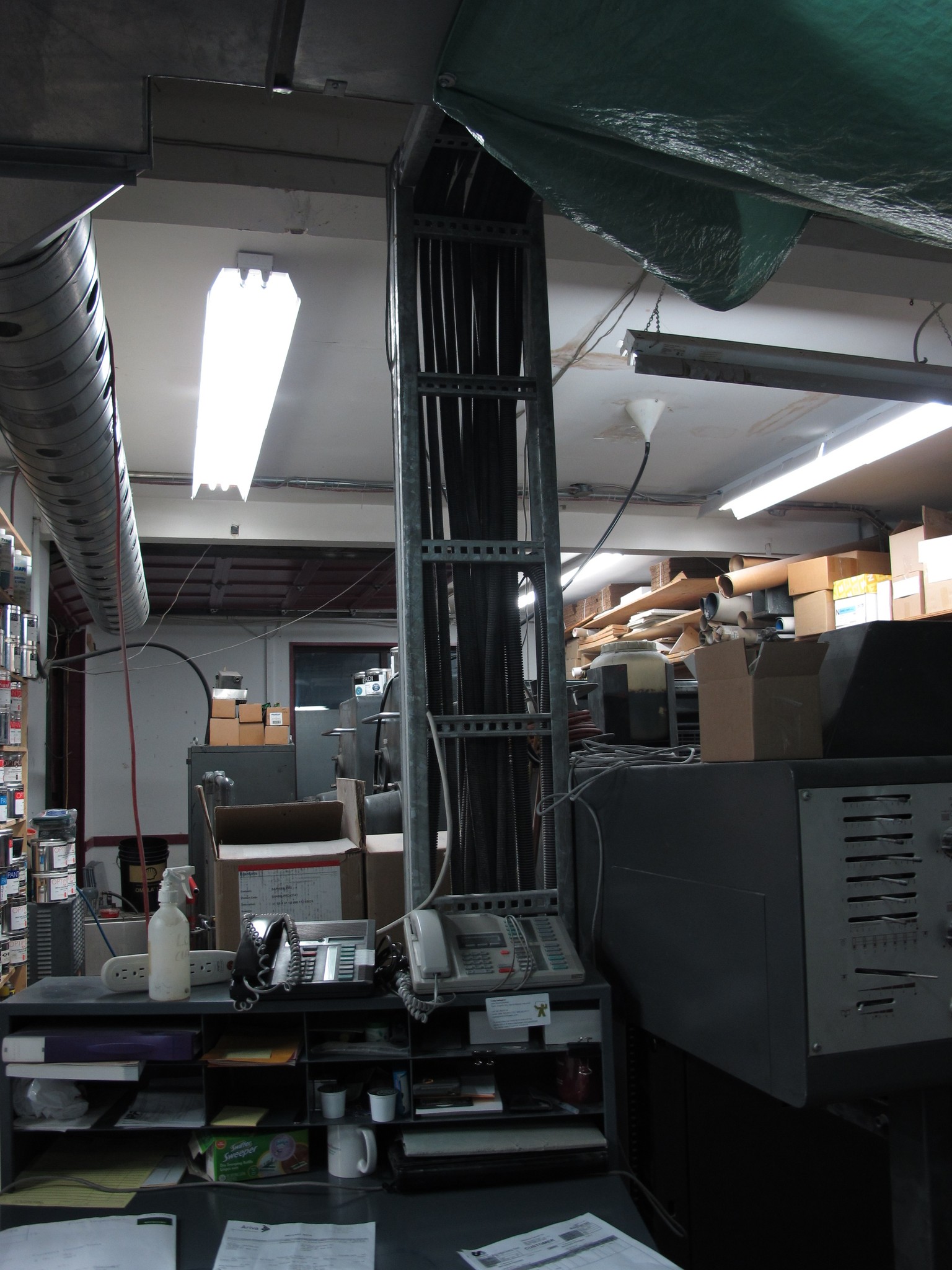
[552,1099,579,1114]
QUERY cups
[326,1125,377,1178]
[367,1087,399,1122]
[317,1083,348,1118]
[365,1027,390,1042]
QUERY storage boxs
[786,506,952,636]
[694,638,829,762]
[210,699,290,745]
[186,1127,310,1183]
[213,779,451,951]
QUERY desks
[84,907,157,975]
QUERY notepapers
[210,1105,269,1126]
[226,1044,273,1058]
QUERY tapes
[366,1023,389,1042]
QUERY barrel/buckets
[0,603,77,966]
[350,667,392,698]
[389,647,400,677]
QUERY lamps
[696,394,952,520]
[191,251,302,502]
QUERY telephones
[229,913,375,1011]
[403,909,586,994]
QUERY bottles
[589,640,671,741]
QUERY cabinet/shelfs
[188,746,297,912]
[0,507,33,1001]
[0,976,624,1184]
